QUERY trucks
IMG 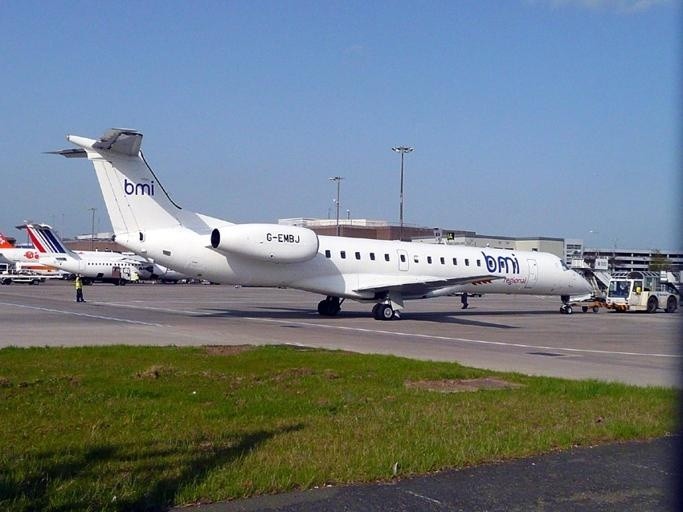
[605,279,681,312]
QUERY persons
[75,276,87,302]
[461,292,468,308]
